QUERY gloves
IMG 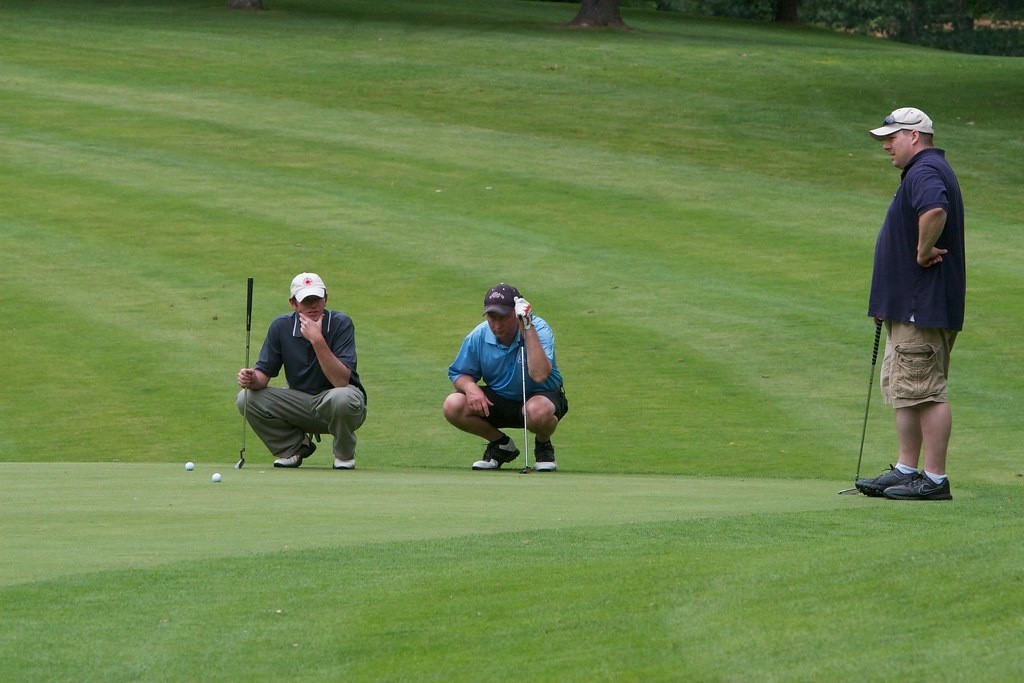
[513,296,535,330]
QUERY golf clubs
[517,294,532,474]
[233,276,255,470]
[838,316,885,496]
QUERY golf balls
[185,461,194,471]
[212,473,221,482]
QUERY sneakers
[274,441,317,467]
[333,455,358,470]
[534,437,558,472]
[855,468,954,500]
[472,436,520,470]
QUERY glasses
[882,116,923,126]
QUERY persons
[856,106,966,501]
[236,273,367,470]
[442,284,569,472]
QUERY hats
[289,271,327,302]
[868,106,935,142]
[483,283,520,317]
